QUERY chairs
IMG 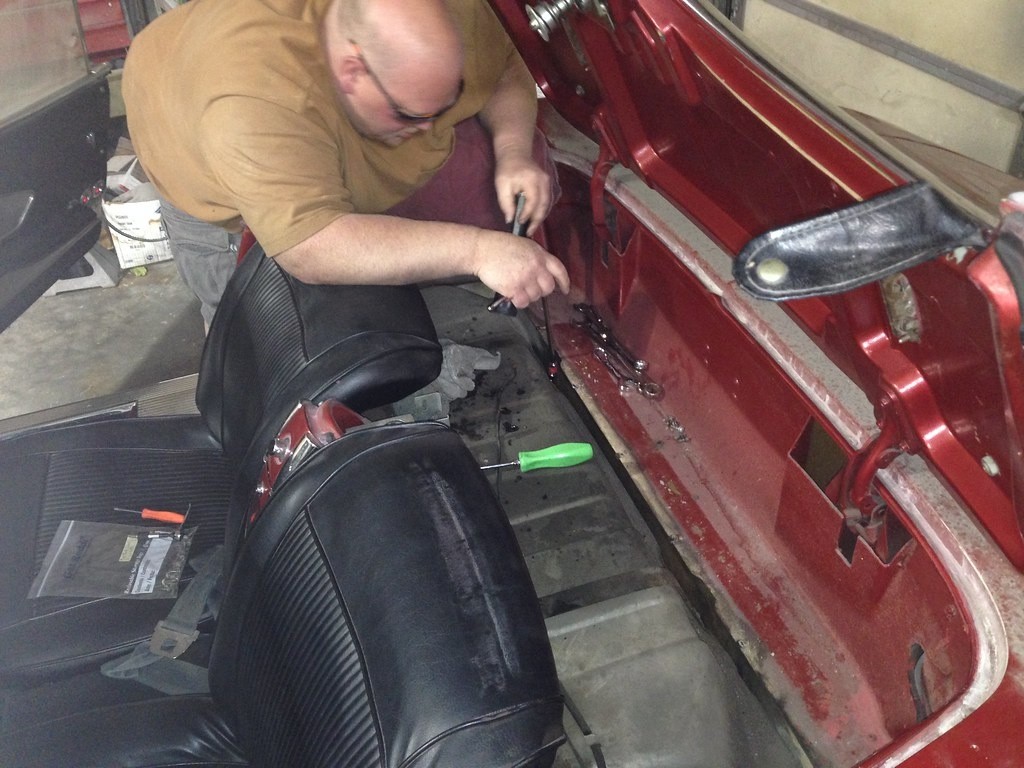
[0,245,566,768]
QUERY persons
[119,0,572,337]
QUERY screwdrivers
[480,441,594,478]
[113,505,185,525]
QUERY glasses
[348,38,464,123]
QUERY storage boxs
[102,162,173,270]
